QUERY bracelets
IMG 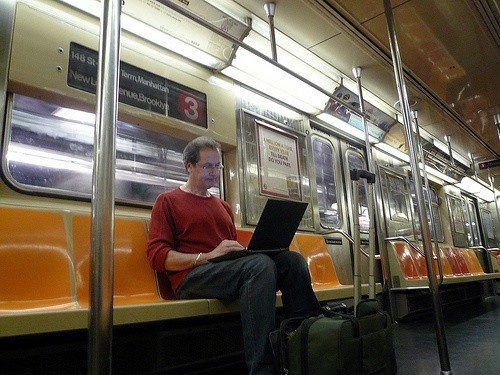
[193,253,203,267]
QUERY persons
[146,136,322,375]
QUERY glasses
[194,163,225,171]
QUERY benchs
[388,241,500,289]
[0,200,384,336]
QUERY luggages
[272,168,397,375]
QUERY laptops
[208,198,309,263]
